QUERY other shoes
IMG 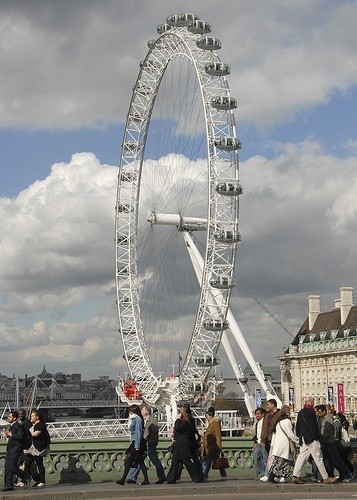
[322,477,337,484]
[309,476,322,483]
[343,472,354,482]
[14,482,24,487]
[155,478,166,484]
[260,475,269,482]
[32,482,40,487]
[292,476,304,484]
[115,479,124,485]
[1,486,14,491]
[274,477,285,482]
[138,480,150,485]
[37,483,45,487]
[125,479,137,484]
[167,481,176,484]
[192,479,204,484]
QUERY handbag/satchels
[289,440,296,454]
[211,455,230,470]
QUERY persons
[1,412,32,491]
[308,402,357,483]
[293,396,337,484]
[166,400,205,484]
[115,405,150,486]
[251,398,299,482]
[200,407,228,480]
[13,409,50,487]
[125,405,167,484]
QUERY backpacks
[334,418,350,442]
